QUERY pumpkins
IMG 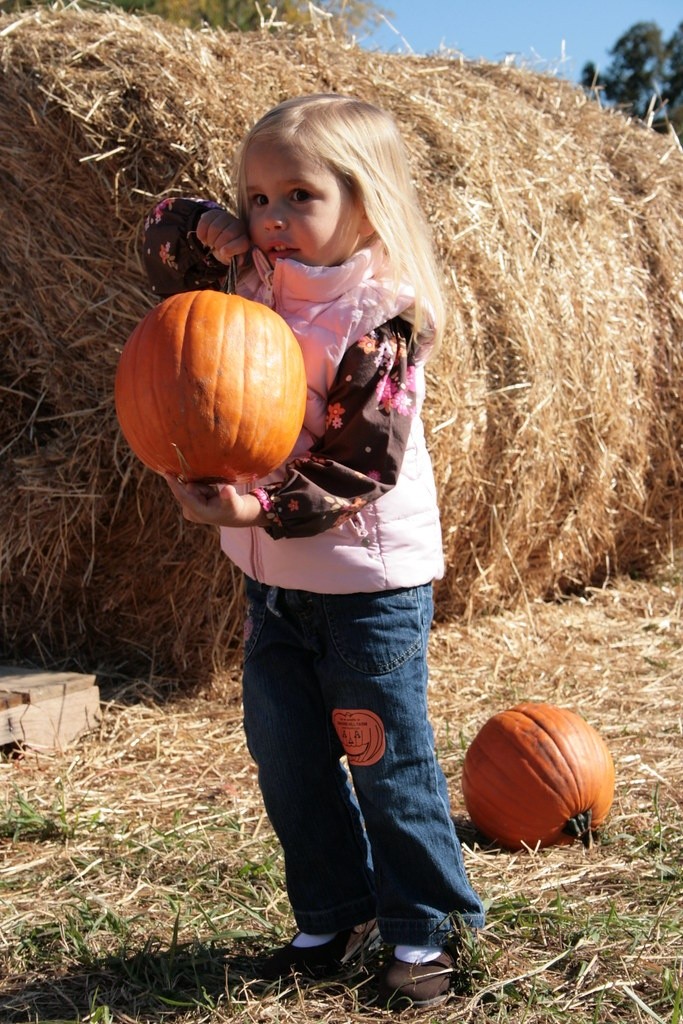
[462,702,616,844]
[117,291,306,484]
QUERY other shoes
[376,941,459,1008]
[267,915,382,982]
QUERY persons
[123,93,491,1008]
